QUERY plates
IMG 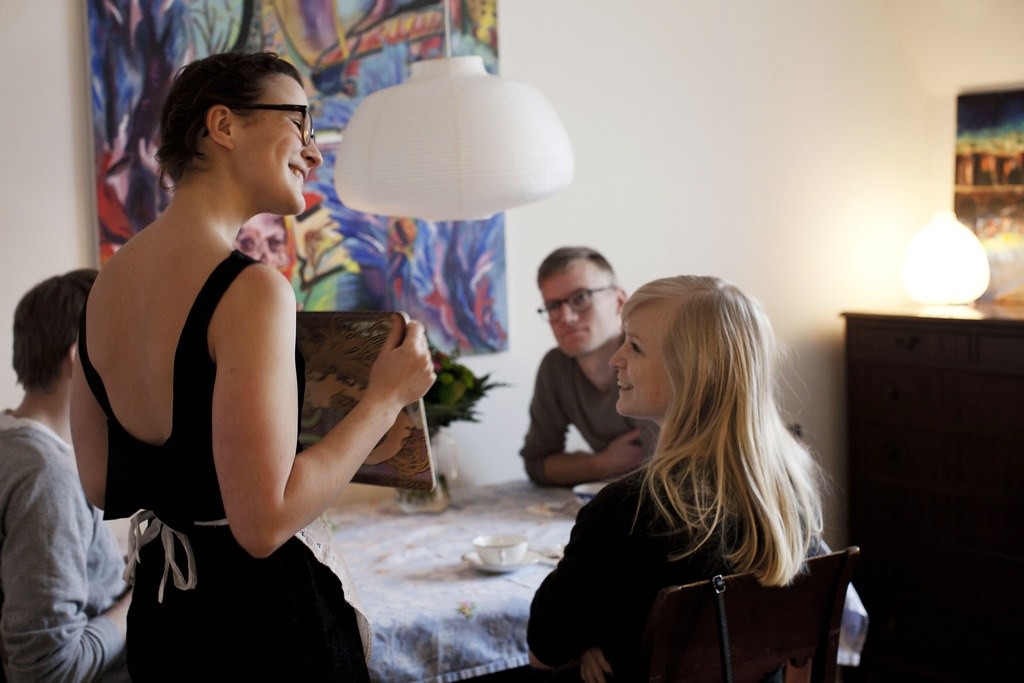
[461,549,541,574]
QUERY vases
[394,427,453,513]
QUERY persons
[518,246,660,490]
[71,51,435,683]
[0,268,132,683]
[526,273,823,683]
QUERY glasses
[537,287,619,321]
[202,103,317,146]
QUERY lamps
[332,0,576,222]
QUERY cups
[573,483,607,506]
[472,534,530,564]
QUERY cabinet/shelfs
[839,311,1024,683]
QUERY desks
[321,479,870,683]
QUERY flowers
[422,333,504,437]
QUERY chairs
[647,546,862,683]
